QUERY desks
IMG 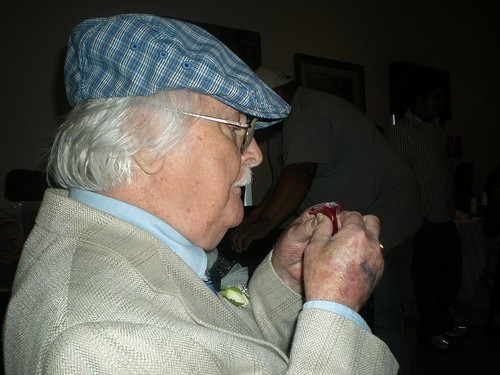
[454,216,500,327]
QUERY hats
[64,14,291,129]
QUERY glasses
[145,102,257,154]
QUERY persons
[2,13,386,375]
[245,108,303,244]
[386,76,468,351]
[229,66,416,375]
[453,163,500,331]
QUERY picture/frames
[165,14,262,75]
[292,52,367,114]
[387,61,455,122]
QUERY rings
[379,243,384,249]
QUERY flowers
[210,256,250,312]
